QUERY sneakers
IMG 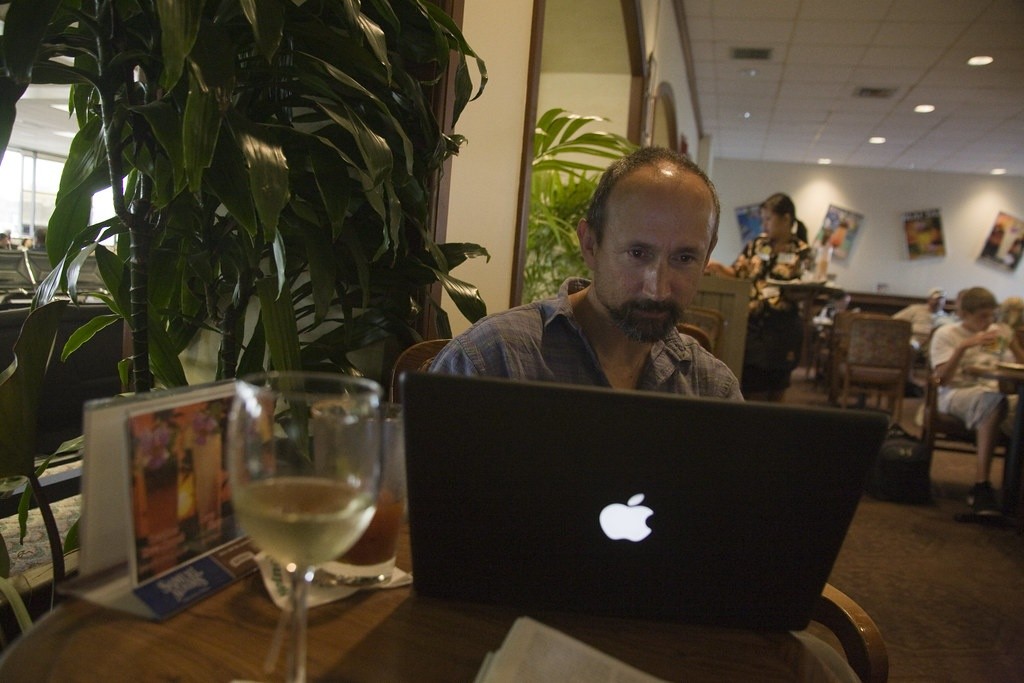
[966,479,1003,518]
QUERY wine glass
[225,371,388,683]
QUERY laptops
[400,372,889,631]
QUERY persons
[893,286,952,398]
[428,145,748,404]
[930,287,1024,522]
[703,190,815,401]
[0,228,47,253]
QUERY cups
[306,402,404,588]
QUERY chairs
[0,301,69,593]
[799,307,1009,502]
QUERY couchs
[0,303,124,454]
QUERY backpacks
[743,298,803,395]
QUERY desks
[0,492,842,683]
[962,368,1024,525]
